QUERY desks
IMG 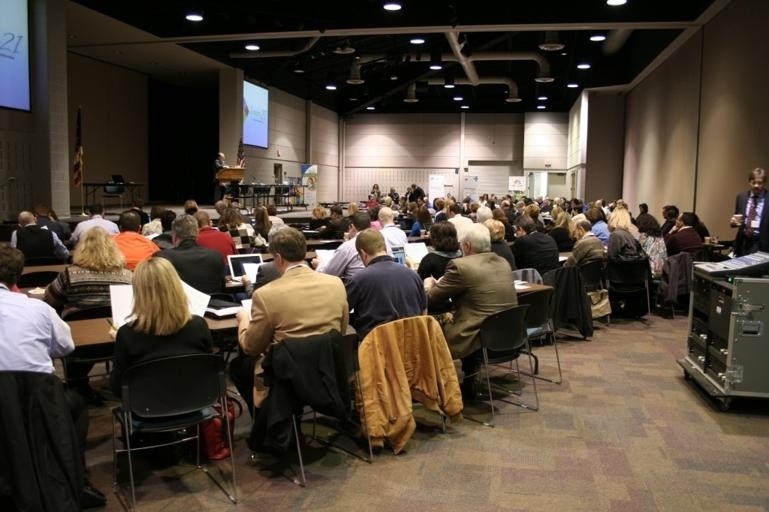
[83,182,149,209]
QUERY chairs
[19,222,447,511]
[578,237,725,327]
[460,245,573,428]
[101,185,125,219]
[226,184,310,215]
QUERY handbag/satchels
[585,289,612,321]
[197,399,235,461]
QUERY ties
[744,194,761,237]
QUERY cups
[733,214,744,226]
[419,229,426,238]
[705,237,710,244]
[343,233,350,242]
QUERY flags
[236,137,245,169]
[72,107,83,188]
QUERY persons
[215,152,231,195]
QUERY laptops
[112,175,125,184]
[227,253,263,282]
